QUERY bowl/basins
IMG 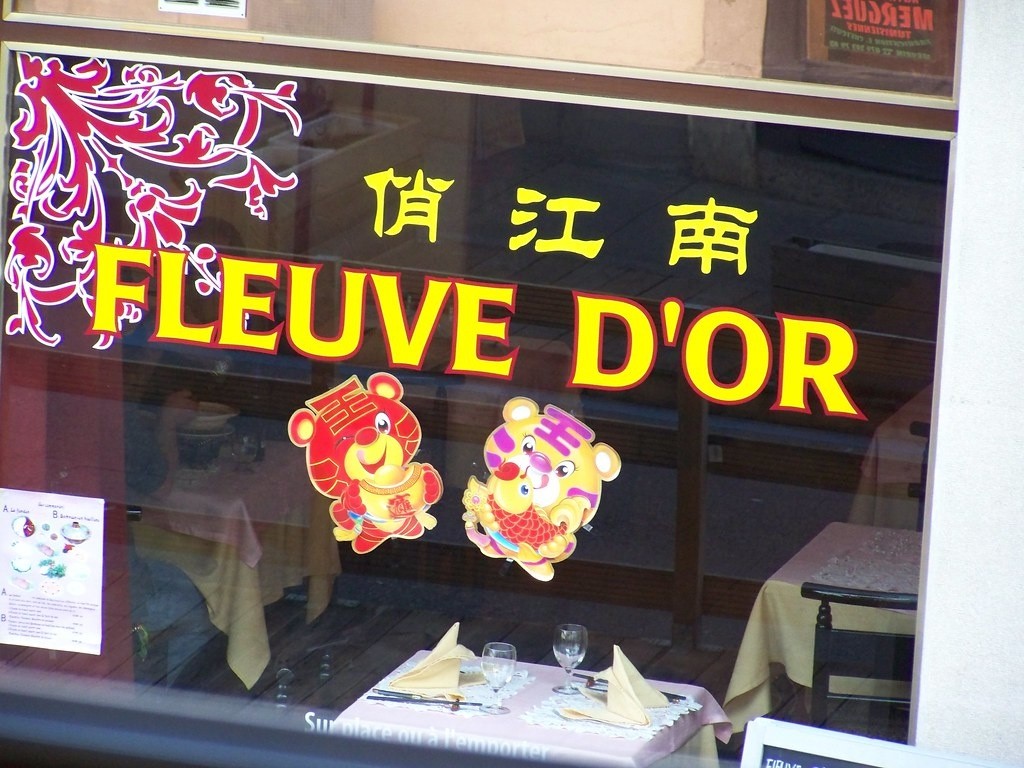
[167,425,236,467]
[162,402,239,430]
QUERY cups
[231,431,257,475]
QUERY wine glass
[552,624,588,695]
[481,642,516,714]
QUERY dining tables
[724,521,922,744]
[336,648,732,768]
[845,377,934,533]
[134,436,343,690]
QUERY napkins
[388,621,485,704]
[557,644,670,729]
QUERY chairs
[800,581,918,738]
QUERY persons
[98,195,334,691]
